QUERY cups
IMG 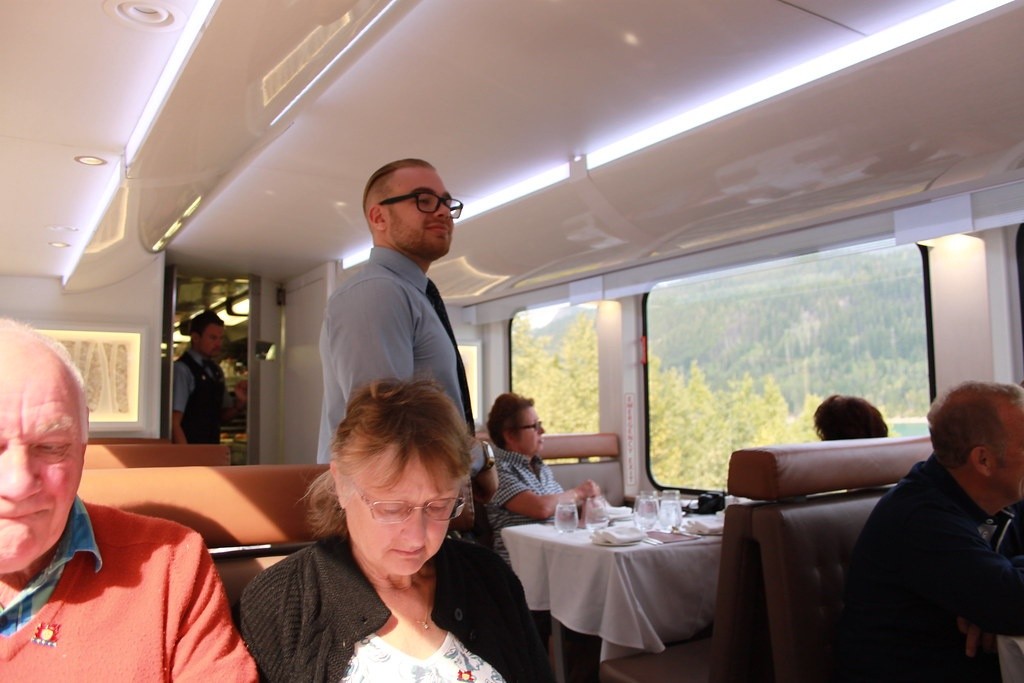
[585,496,609,532]
[662,490,680,501]
[634,495,653,527]
[641,490,658,507]
[554,494,579,535]
[639,499,659,530]
[686,490,725,513]
[660,501,682,530]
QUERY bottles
[234,362,242,375]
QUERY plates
[610,512,633,521]
[691,531,723,535]
[593,541,638,546]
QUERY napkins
[587,525,648,544]
[680,514,725,536]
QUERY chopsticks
[644,538,662,546]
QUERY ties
[426,278,475,439]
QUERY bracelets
[483,440,496,471]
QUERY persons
[233,376,544,683]
[317,158,500,545]
[0,318,258,683]
[485,393,599,566]
[841,380,1024,683]
[814,395,888,442]
[173,311,230,444]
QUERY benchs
[79,427,937,682]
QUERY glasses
[379,191,463,219]
[352,482,466,523]
[517,421,542,431]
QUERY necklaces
[388,598,432,630]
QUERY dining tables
[501,507,725,682]
